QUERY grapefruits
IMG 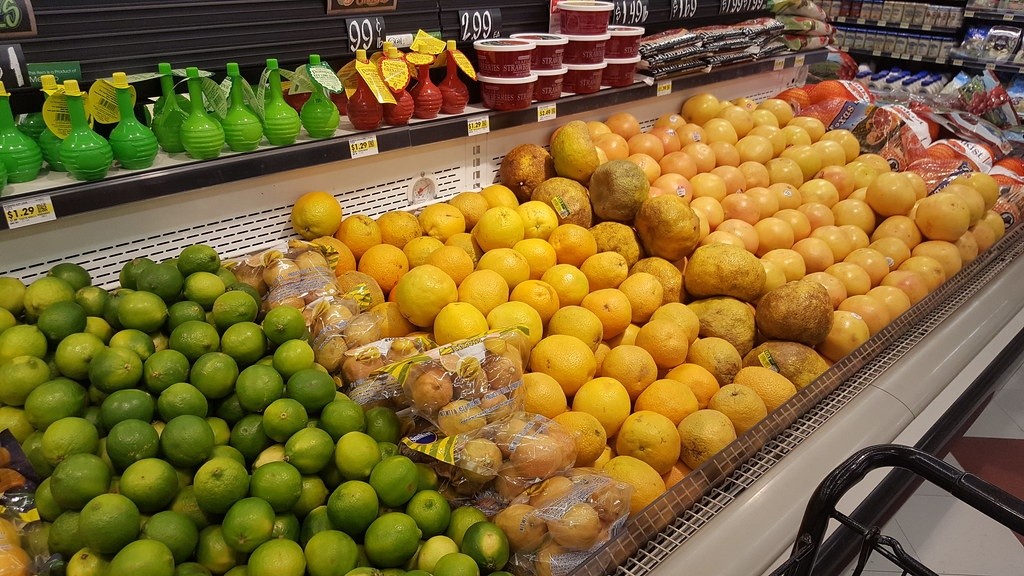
[499,120,833,391]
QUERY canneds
[473,1,645,110]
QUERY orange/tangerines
[3,244,515,576]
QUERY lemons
[237,184,797,574]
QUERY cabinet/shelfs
[0,1,1024,576]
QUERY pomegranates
[586,93,1006,361]
